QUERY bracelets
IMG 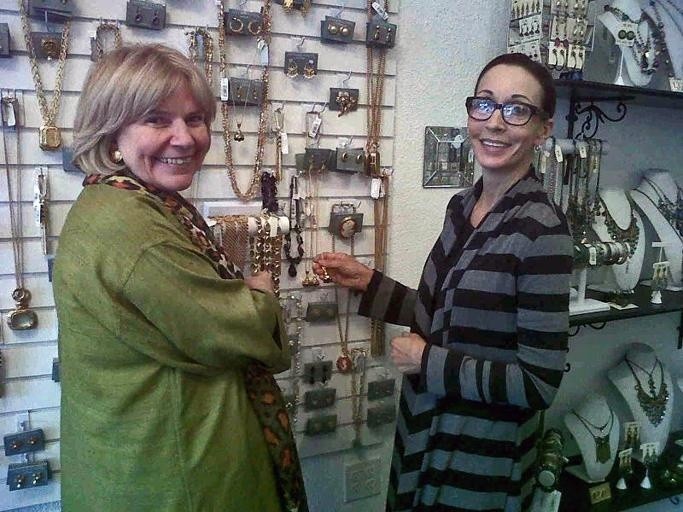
[535,432,567,493]
[573,237,629,268]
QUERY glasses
[466,97,550,126]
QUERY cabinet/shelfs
[545,77,683,512]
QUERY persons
[310,50,576,512]
[49,43,310,512]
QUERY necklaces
[1,95,39,331]
[301,176,333,289]
[365,45,386,148]
[283,174,304,277]
[224,43,260,142]
[540,136,683,286]
[18,0,71,152]
[277,293,305,423]
[94,23,123,62]
[217,3,272,202]
[348,348,367,449]
[371,177,389,362]
[215,210,282,300]
[572,347,683,469]
[605,1,683,84]
[330,228,355,374]
[187,28,214,88]
